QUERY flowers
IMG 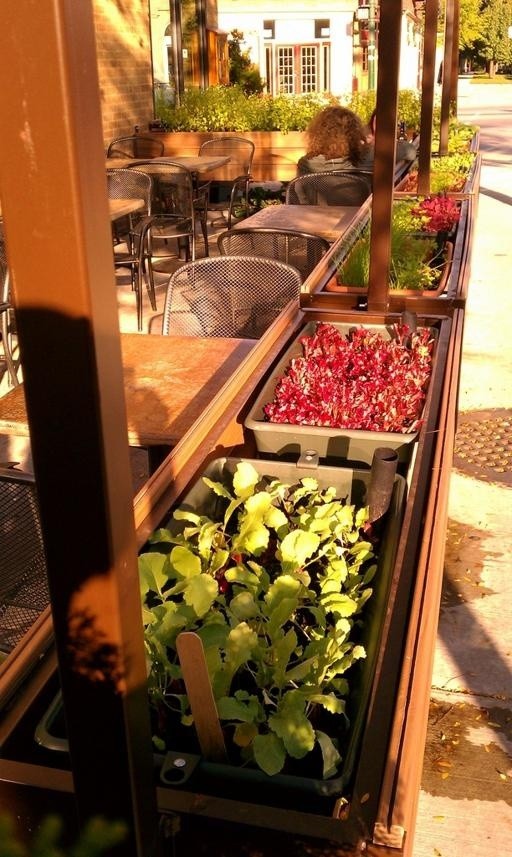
[392,195,461,232]
[262,323,433,433]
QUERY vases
[366,199,457,238]
[246,323,416,461]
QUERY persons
[361,111,377,143]
[295,107,417,207]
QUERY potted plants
[393,139,475,195]
[326,237,453,296]
[34,458,406,799]
